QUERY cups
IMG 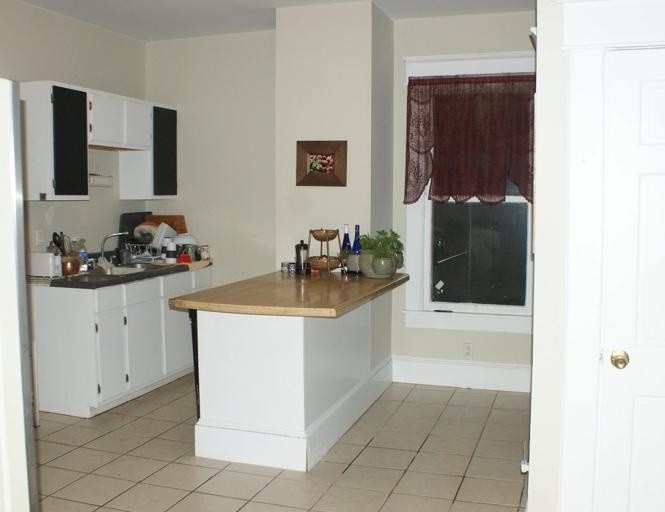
[281,262,295,273]
[161,237,210,265]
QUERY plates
[133,220,160,241]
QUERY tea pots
[295,239,311,275]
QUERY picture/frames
[296,140,347,186]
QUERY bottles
[341,224,352,275]
[71,238,89,271]
[352,225,361,274]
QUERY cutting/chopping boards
[145,215,187,233]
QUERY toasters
[27,252,62,277]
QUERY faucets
[101,231,129,259]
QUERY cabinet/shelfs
[20,81,177,200]
[25,266,212,419]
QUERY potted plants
[339,230,404,279]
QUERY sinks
[116,263,165,269]
